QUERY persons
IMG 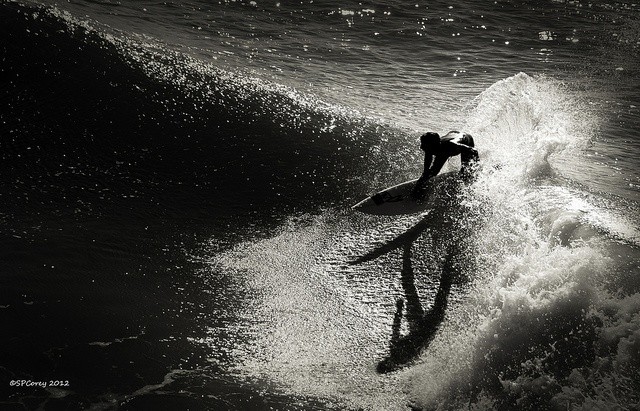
[418,128,478,181]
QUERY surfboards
[352,169,463,216]
